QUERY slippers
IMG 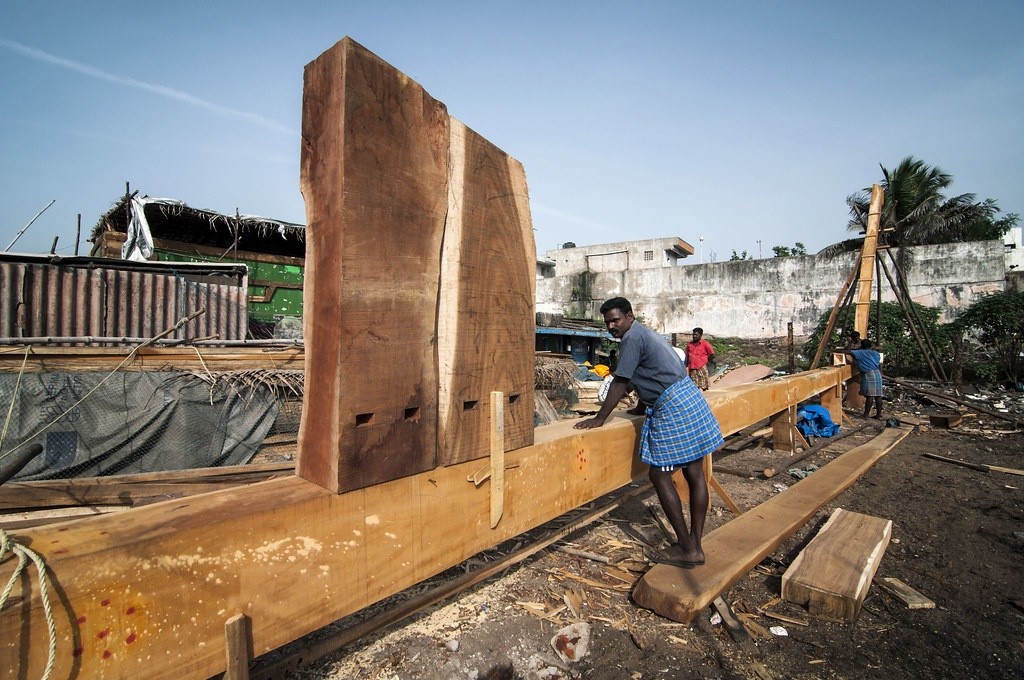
[644,549,704,568]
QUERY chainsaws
[886,416,900,428]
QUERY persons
[574,297,724,568]
[833,339,882,419]
[684,328,716,390]
[846,331,863,350]
